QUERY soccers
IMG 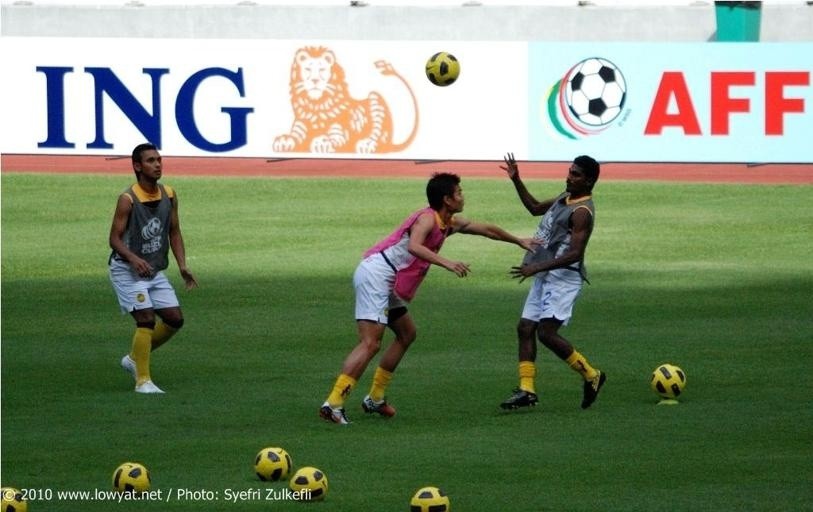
[111,462,150,497]
[1,487,27,512]
[563,58,627,124]
[291,466,328,501]
[649,363,686,399]
[254,447,291,480]
[410,486,449,512]
[424,51,459,86]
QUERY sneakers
[119,355,137,374]
[318,401,350,424]
[581,368,606,409]
[135,380,166,393]
[360,395,396,417]
[499,384,539,411]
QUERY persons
[499,152,606,411]
[107,143,200,394]
[320,172,546,425]
